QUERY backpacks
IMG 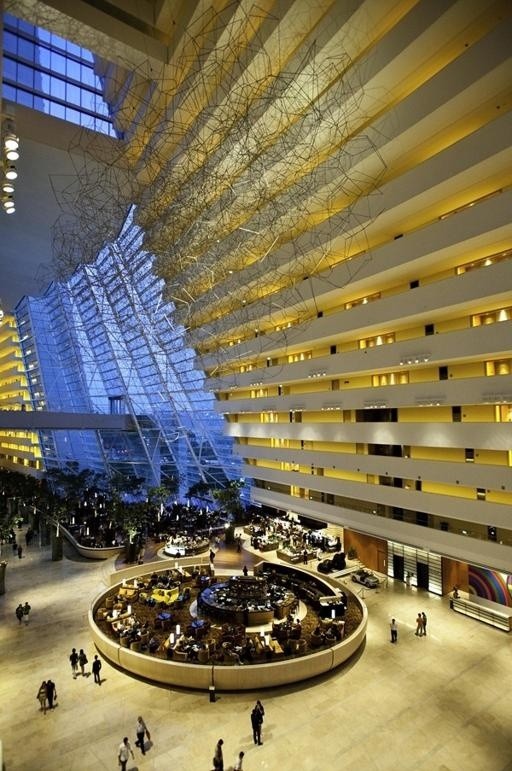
[214,757,220,768]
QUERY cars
[350,568,379,587]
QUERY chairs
[98,564,345,665]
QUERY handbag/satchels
[37,694,39,699]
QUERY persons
[388,620,398,644]
[255,701,264,735]
[213,750,223,771]
[135,716,150,756]
[22,602,31,627]
[250,708,262,746]
[16,603,22,625]
[79,649,89,678]
[234,752,244,771]
[47,681,57,710]
[8,521,35,559]
[92,655,103,685]
[106,513,341,664]
[213,740,224,753]
[37,682,48,715]
[69,648,79,679]
[414,614,422,638]
[117,737,136,771]
[421,613,426,635]
